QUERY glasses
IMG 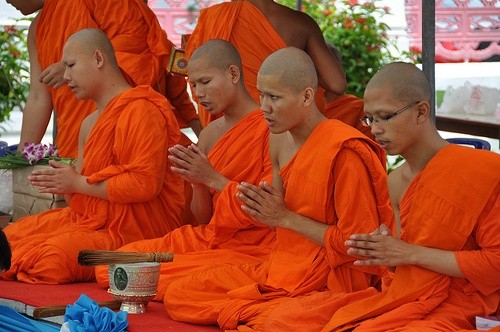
[359,100,421,127]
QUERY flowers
[0,139,73,170]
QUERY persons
[95,39,273,303]
[260,62,499,332]
[142,0,202,138]
[0,27,197,284]
[4,0,173,158]
[163,47,398,332]
[183,1,376,130]
